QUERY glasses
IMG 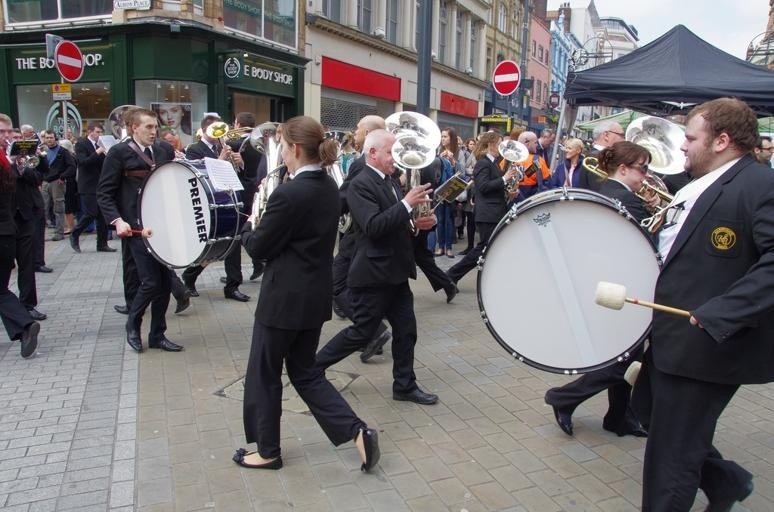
[629,163,649,175]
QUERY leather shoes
[544,391,574,435]
[174,280,199,313]
[444,282,456,303]
[115,305,128,314]
[29,308,48,320]
[703,480,753,511]
[69,232,81,252]
[361,428,380,471]
[220,276,250,300]
[148,338,183,352]
[393,389,439,405]
[233,447,283,470]
[36,265,53,273]
[359,330,392,362]
[602,414,648,437]
[125,324,142,351]
[97,244,116,252]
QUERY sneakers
[51,232,63,241]
[20,321,40,357]
[434,248,471,258]
[250,264,264,280]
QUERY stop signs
[490,60,522,97]
[52,39,85,83]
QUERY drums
[477,188,663,376]
[138,159,244,270]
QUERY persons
[642,98,773,511]
[0,108,558,362]
[315,129,439,404]
[232,117,380,473]
[156,104,192,147]
[544,115,774,437]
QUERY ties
[212,143,217,158]
[144,147,153,163]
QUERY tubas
[323,131,352,233]
[625,116,687,214]
[250,121,287,232]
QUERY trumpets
[3,137,49,170]
[207,121,240,173]
[391,137,436,235]
[227,127,254,139]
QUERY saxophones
[498,139,529,205]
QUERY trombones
[582,156,674,211]
